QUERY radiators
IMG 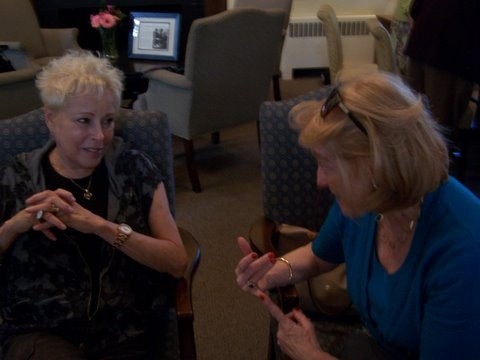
[282,15,377,69]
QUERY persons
[0,49,189,360]
[235,68,480,360]
[402,0,480,130]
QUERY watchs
[112,224,132,248]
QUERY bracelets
[279,257,292,283]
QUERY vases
[99,29,120,61]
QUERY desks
[121,57,185,109]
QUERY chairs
[316,5,397,85]
[0,102,202,360]
[131,7,287,193]
[0,0,79,118]
[249,85,431,360]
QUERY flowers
[89,0,127,52]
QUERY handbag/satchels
[248,218,357,317]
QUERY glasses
[320,88,368,138]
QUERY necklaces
[70,178,92,200]
[376,214,413,230]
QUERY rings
[36,210,43,219]
[52,205,58,212]
[247,281,256,287]
